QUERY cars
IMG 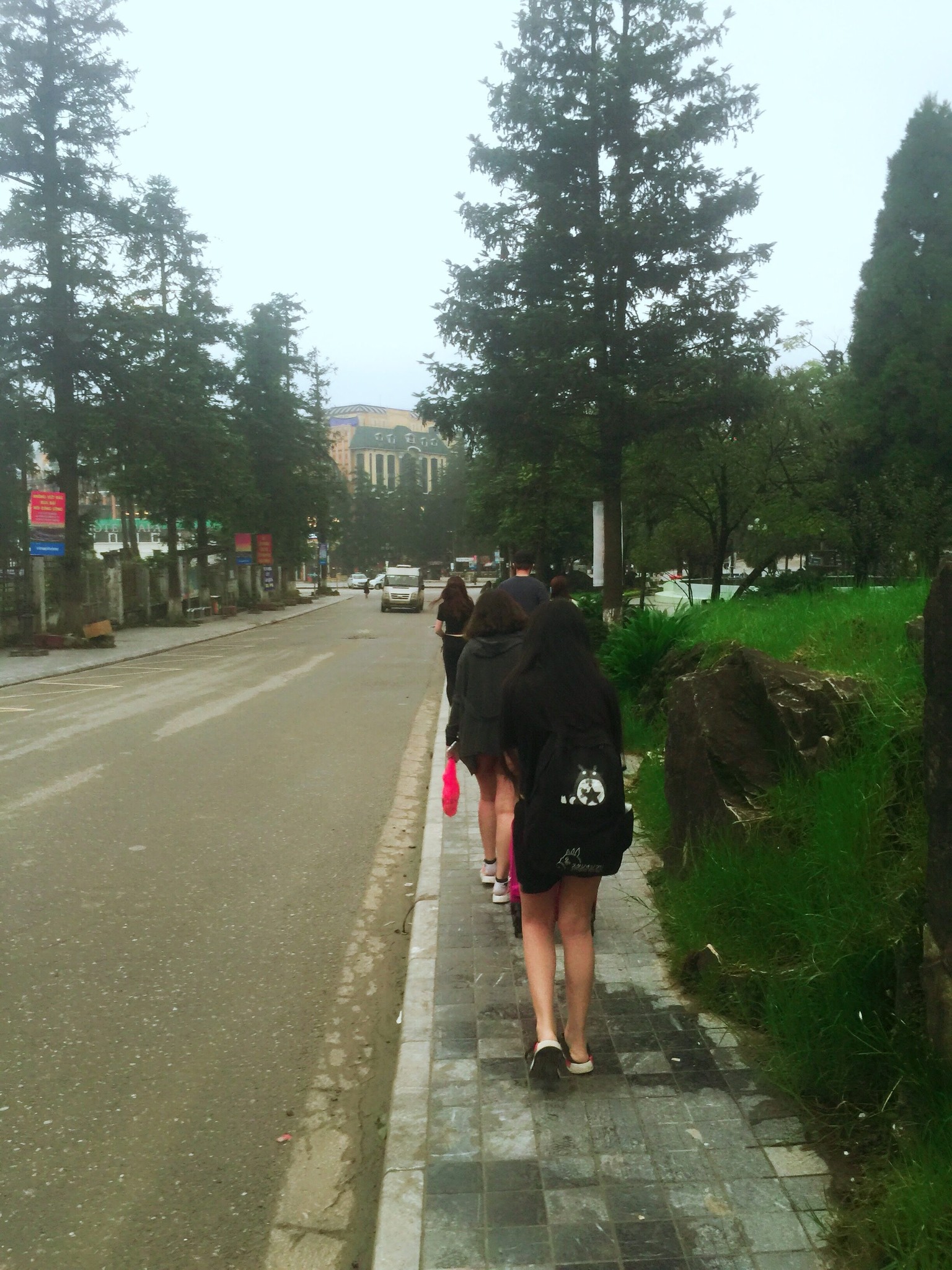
[367,574,386,590]
[348,573,370,589]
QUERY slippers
[561,1031,595,1073]
[480,865,496,883]
[492,888,511,903]
[527,1041,563,1081]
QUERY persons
[499,597,624,1079]
[364,579,370,598]
[445,589,529,903]
[480,581,491,594]
[436,577,474,708]
[550,575,572,600]
[498,550,547,616]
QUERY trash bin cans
[669,574,682,580]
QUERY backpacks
[519,670,636,878]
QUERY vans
[378,564,425,613]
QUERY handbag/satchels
[443,756,460,816]
